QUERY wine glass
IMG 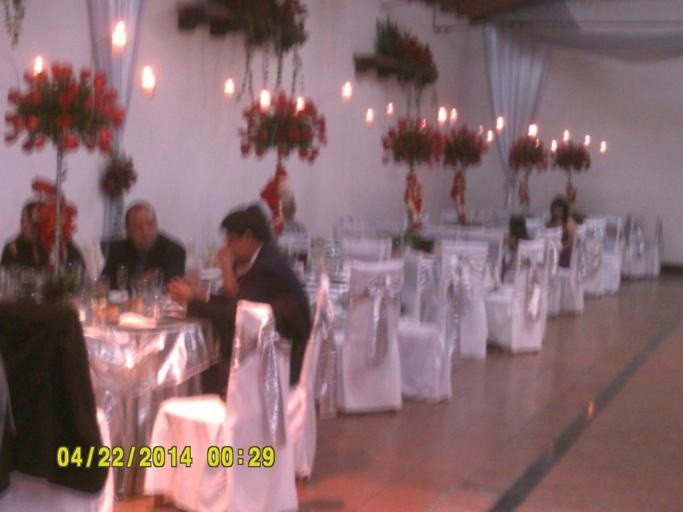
[1,260,189,328]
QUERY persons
[545,197,576,267]
[167,206,312,387]
[96,204,184,292]
[0,202,86,271]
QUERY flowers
[3,59,127,270]
[379,119,594,230]
[239,95,330,239]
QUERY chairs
[0,208,666,512]
